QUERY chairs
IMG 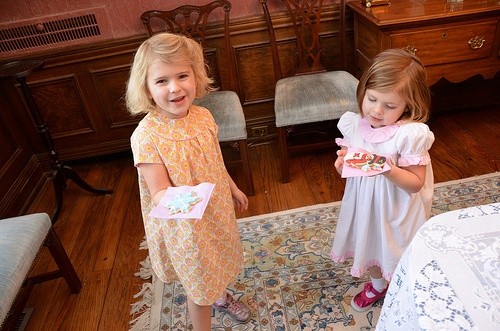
[257,0,358,184]
[0,213,81,331]
[139,0,255,197]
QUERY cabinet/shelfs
[347,0,500,119]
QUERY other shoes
[350,282,389,312]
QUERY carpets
[129,171,500,331]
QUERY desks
[374,202,500,331]
[0,58,113,224]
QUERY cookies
[165,190,202,215]
[344,153,386,172]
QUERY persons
[124,33,252,331]
[331,49,435,312]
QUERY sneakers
[212,292,251,324]
